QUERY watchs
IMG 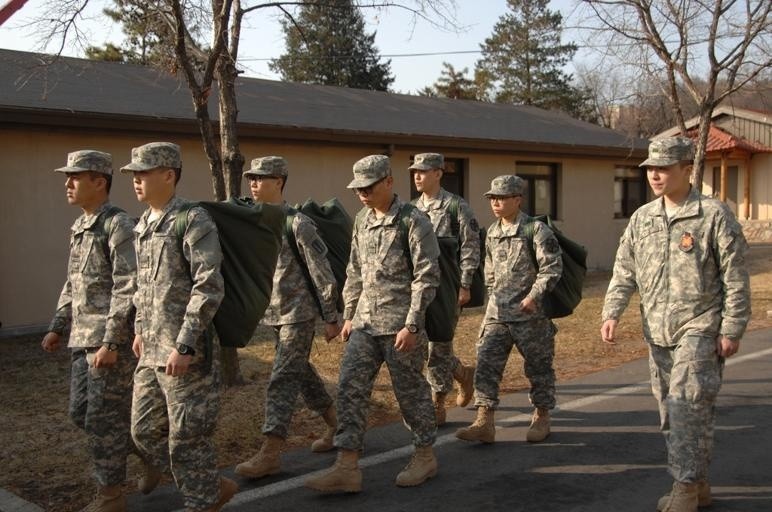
[175,342,195,357]
[404,323,420,334]
[103,342,120,351]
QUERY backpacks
[527,214,587,318]
[450,194,486,307]
[176,197,282,348]
[399,203,458,341]
[286,197,351,319]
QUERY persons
[600,133,751,512]
[405,153,482,430]
[233,155,342,479]
[302,151,439,493]
[40,149,164,511]
[120,141,238,512]
[454,174,568,444]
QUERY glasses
[487,197,512,202]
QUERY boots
[235,434,282,478]
[81,482,128,512]
[395,444,438,487]
[526,407,550,442]
[311,405,338,452]
[454,360,476,406]
[432,391,446,426]
[456,407,495,443]
[657,479,711,512]
[215,475,238,510]
[133,449,162,493]
[305,446,362,492]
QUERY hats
[639,137,695,167]
[346,154,392,189]
[408,153,444,171]
[54,150,113,175]
[483,175,525,196]
[120,142,182,174]
[242,156,289,178]
